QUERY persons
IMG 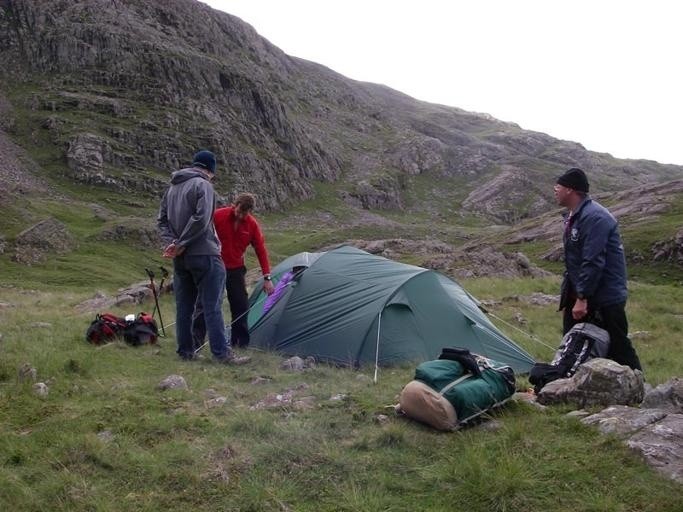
[553,168,643,372]
[157,150,250,365]
[192,191,274,348]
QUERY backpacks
[551,322,609,377]
[87,314,126,344]
[538,357,644,407]
[125,312,158,346]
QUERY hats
[557,167,590,192]
[194,150,216,173]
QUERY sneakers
[225,355,251,365]
[181,354,205,360]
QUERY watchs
[262,274,272,282]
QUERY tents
[231,244,544,377]
